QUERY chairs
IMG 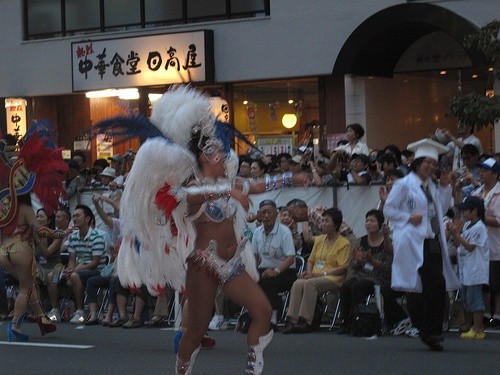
[231,256,304,331]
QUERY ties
[420,183,440,236]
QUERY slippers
[460,326,487,340]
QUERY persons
[0,134,175,341]
[209,123,418,334]
[432,130,500,338]
[384,139,461,350]
[176,125,314,375]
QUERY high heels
[174,328,215,354]
[38,314,56,335]
[6,322,30,342]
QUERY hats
[289,156,304,166]
[406,137,450,165]
[476,157,500,171]
[458,196,482,211]
[352,153,370,165]
[99,167,117,180]
[108,154,122,164]
[259,200,276,209]
[332,149,349,163]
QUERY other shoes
[85,315,113,326]
[0,307,37,322]
[282,319,313,333]
[442,307,500,331]
[142,315,166,325]
[322,311,342,325]
[107,317,143,328]
[336,316,355,336]
[239,316,278,333]
[419,330,446,353]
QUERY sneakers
[208,314,225,330]
[49,308,61,322]
[394,316,420,337]
[69,308,87,324]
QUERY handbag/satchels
[352,312,383,337]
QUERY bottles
[360,261,375,273]
[264,172,293,192]
[460,176,474,195]
